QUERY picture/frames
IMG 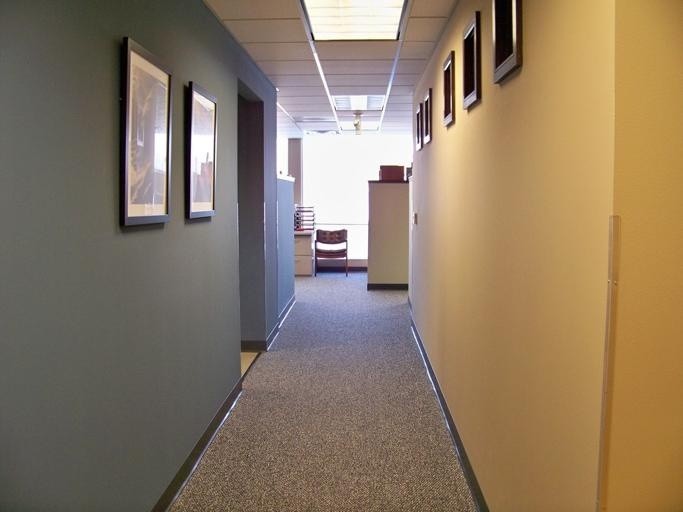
[420,88,432,145]
[461,12,482,111]
[118,37,176,226]
[439,50,454,128]
[491,0,522,85]
[415,110,421,151]
[186,81,219,220]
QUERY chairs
[315,230,349,277]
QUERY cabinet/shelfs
[293,234,313,276]
